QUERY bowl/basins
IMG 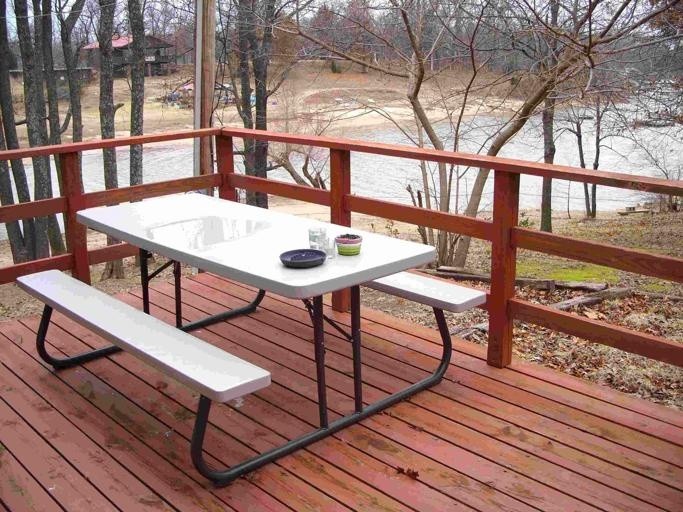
[334,235,362,255]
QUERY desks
[75,193,436,484]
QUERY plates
[279,249,327,267]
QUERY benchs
[359,271,487,383]
[14,269,271,484]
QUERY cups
[309,227,325,249]
[315,237,334,259]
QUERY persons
[161,83,256,115]
